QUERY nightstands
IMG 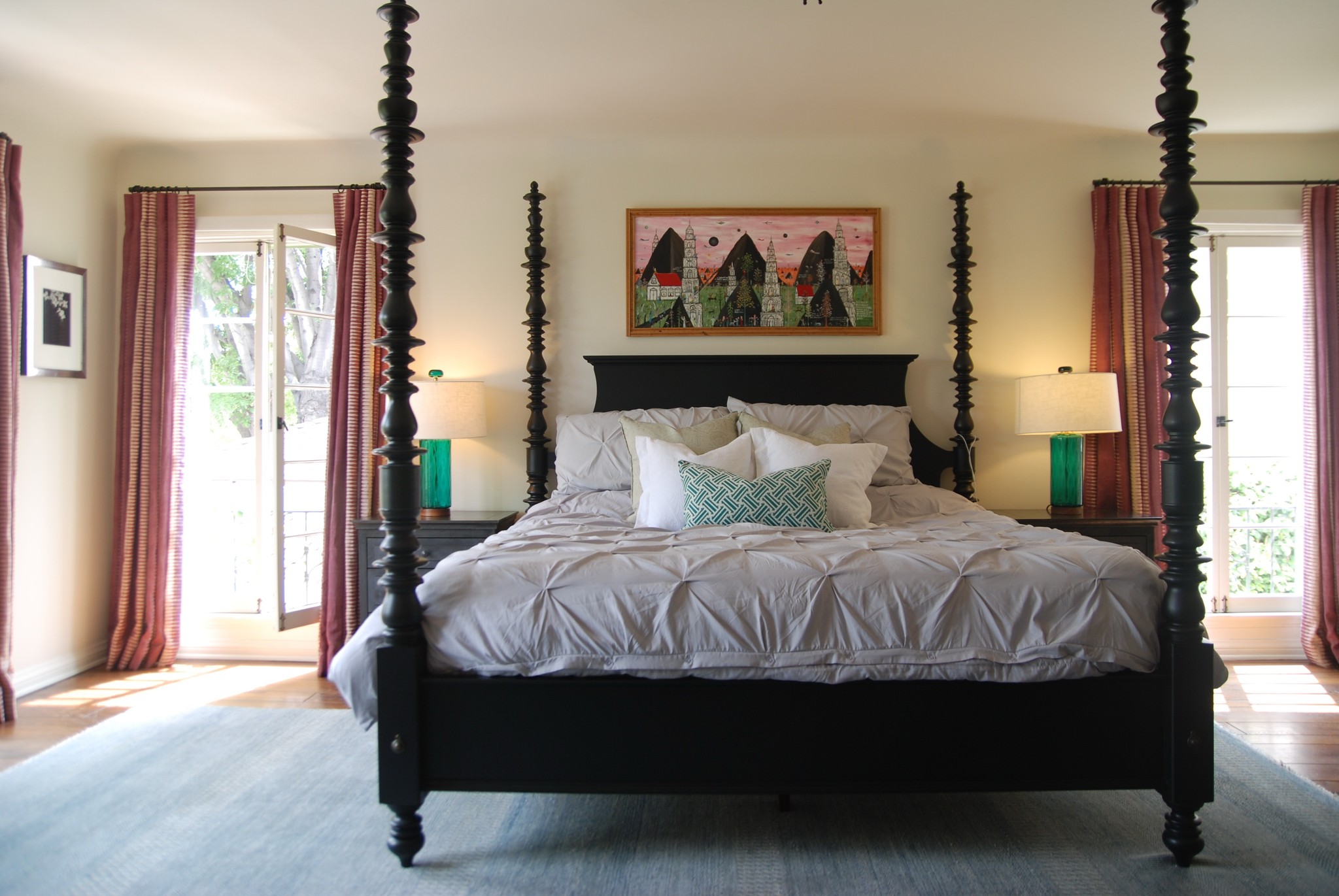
[353,511,520,627]
[987,509,1164,561]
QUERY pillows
[551,396,916,529]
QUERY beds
[328,1,1229,870]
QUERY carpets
[0,694,1339,896]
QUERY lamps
[409,369,487,516]
[1016,366,1123,515]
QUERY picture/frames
[20,254,87,379]
[625,206,883,337]
